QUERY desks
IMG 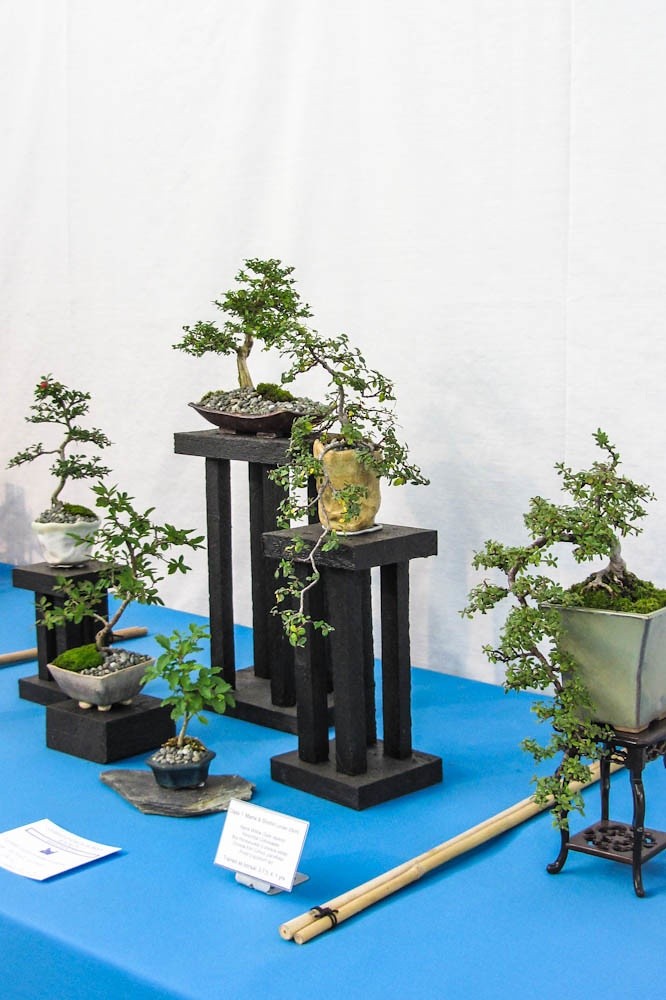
[260,522,442,811]
[546,720,666,898]
[13,560,124,705]
[174,428,345,736]
[0,568,666,1000]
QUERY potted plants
[5,373,114,568]
[139,623,236,788]
[259,321,429,644]
[30,480,207,705]
[169,258,326,437]
[458,426,666,832]
